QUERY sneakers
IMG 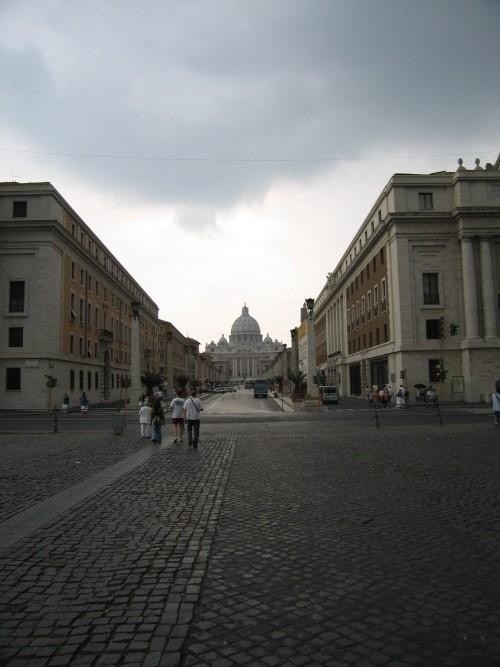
[142,437,198,449]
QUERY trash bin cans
[112,414,126,435]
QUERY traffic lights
[449,323,458,337]
[399,370,404,380]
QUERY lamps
[291,329,297,341]
[129,300,141,320]
[166,330,173,345]
[305,297,315,317]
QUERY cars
[211,385,237,394]
[245,379,268,398]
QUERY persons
[62,391,70,413]
[363,383,439,408]
[492,379,499,429]
[183,389,204,448]
[170,391,184,443]
[137,401,152,438]
[151,399,165,443]
[79,391,89,416]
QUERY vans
[318,385,339,405]
